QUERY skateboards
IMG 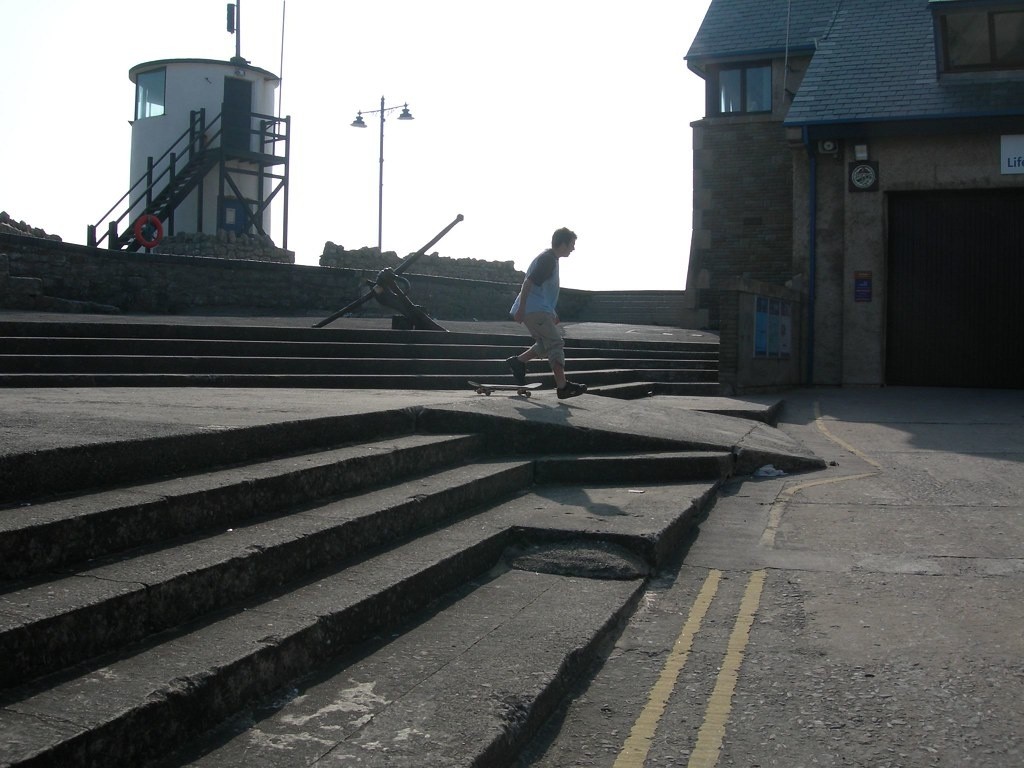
[468,380,542,398]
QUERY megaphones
[819,139,838,153]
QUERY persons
[506,227,588,400]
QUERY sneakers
[506,356,526,383]
[557,380,587,399]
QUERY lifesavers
[135,214,162,247]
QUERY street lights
[350,96,414,251]
